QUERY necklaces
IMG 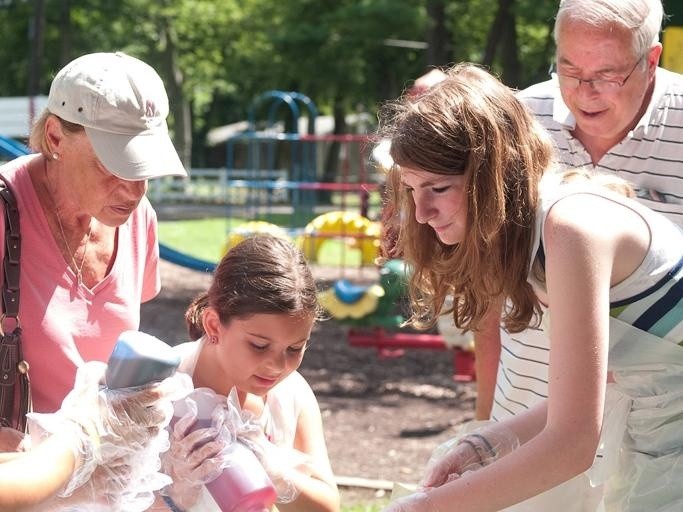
[51,210,99,289]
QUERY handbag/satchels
[1,334,18,428]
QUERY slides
[0,133,216,274]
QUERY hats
[49,52,188,181]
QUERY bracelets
[158,487,186,512]
[466,433,499,461]
[457,437,486,467]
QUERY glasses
[549,59,644,93]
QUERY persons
[0,356,177,511]
[452,0,683,512]
[149,230,344,512]
[369,58,683,512]
[0,46,192,451]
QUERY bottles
[168,385,277,512]
[100,328,181,391]
